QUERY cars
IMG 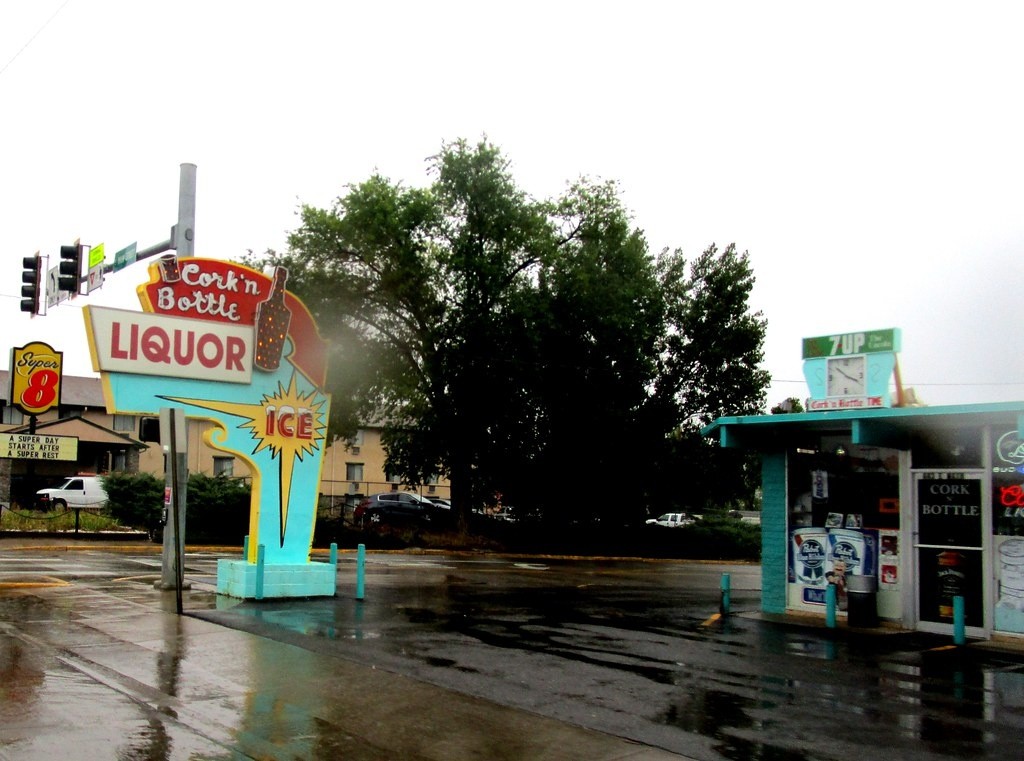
[494,507,519,522]
[429,499,483,515]
[360,492,451,524]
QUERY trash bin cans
[844,575,878,630]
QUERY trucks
[36,476,108,512]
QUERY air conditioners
[427,486,436,493]
[390,484,398,491]
[352,483,360,490]
[351,447,360,455]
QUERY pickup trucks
[646,513,695,527]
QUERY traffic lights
[58,244,82,294]
[21,256,41,314]
